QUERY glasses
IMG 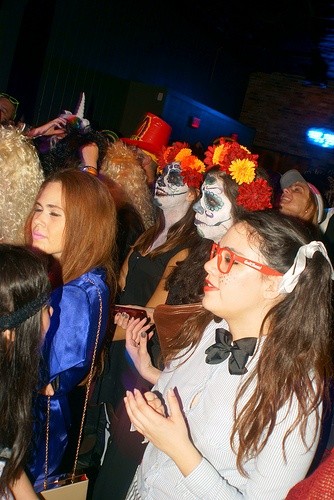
[1,92,19,119]
[210,242,281,279]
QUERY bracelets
[82,167,98,176]
[83,166,97,170]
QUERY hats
[279,168,323,224]
[117,112,173,157]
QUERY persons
[0,94,334,500]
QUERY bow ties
[204,328,258,376]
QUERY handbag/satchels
[37,474,89,500]
